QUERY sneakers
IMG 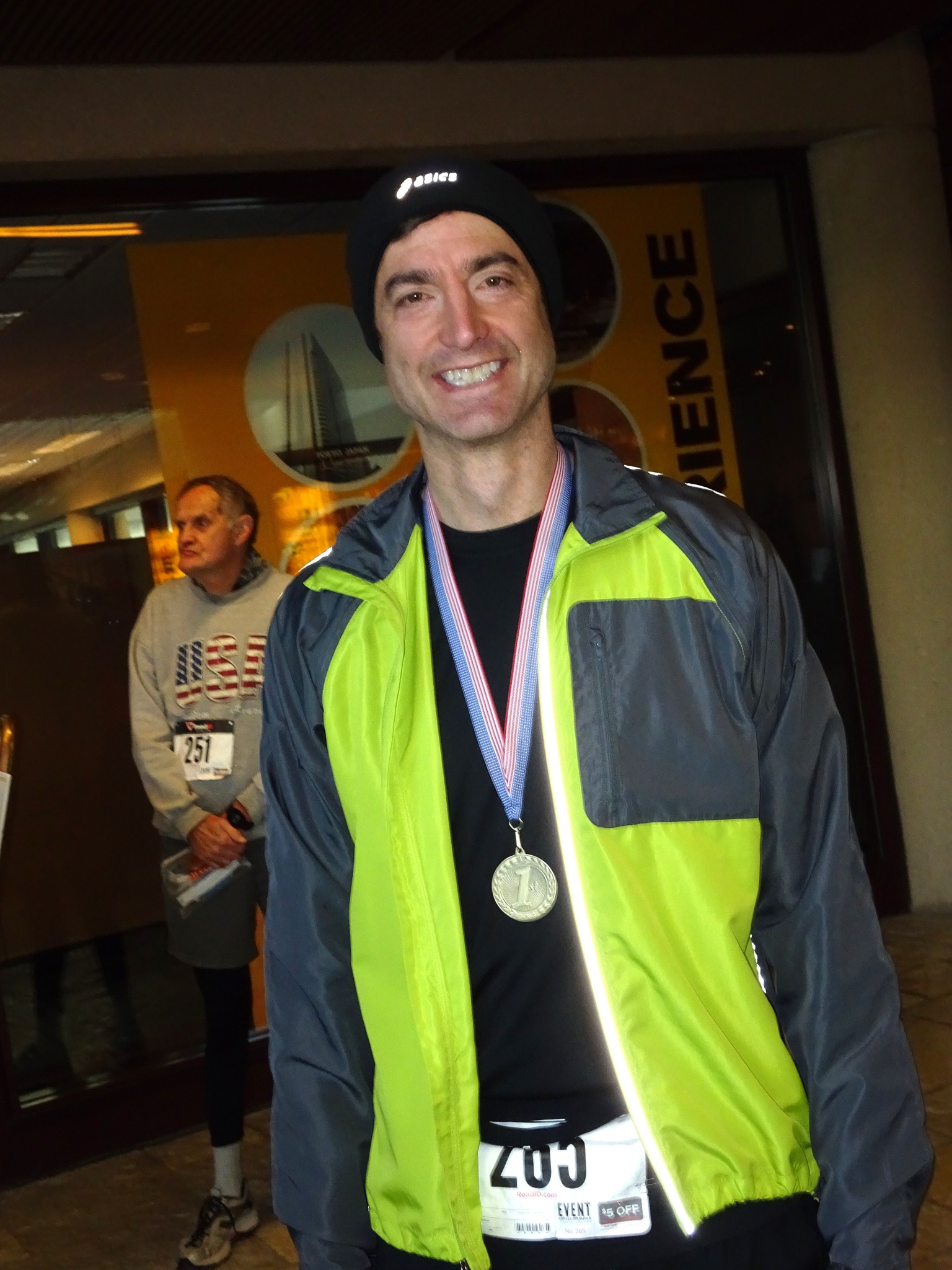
[177,1179,263,1266]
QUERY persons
[127,475,299,1269]
[260,149,935,1270]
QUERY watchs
[222,804,255,833]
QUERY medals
[491,852,557,923]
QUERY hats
[348,162,620,370]
